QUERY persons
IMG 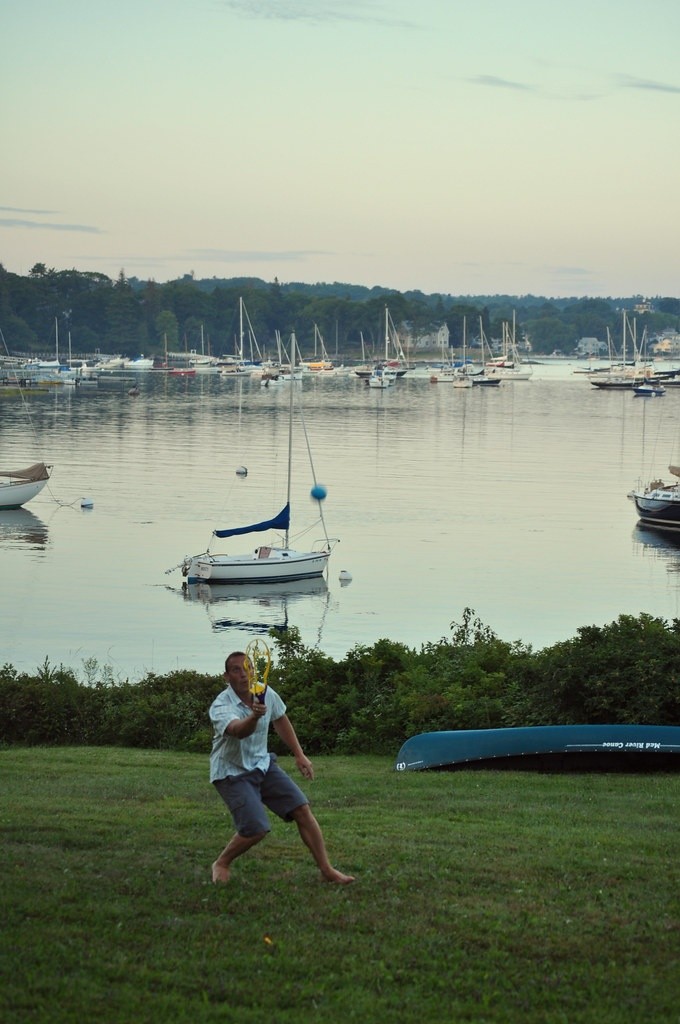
[210,652,355,886]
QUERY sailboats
[165,296,534,390]
[572,309,680,396]
[177,331,331,582]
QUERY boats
[0,315,138,392]
[0,461,55,512]
[389,721,680,773]
[625,463,680,537]
